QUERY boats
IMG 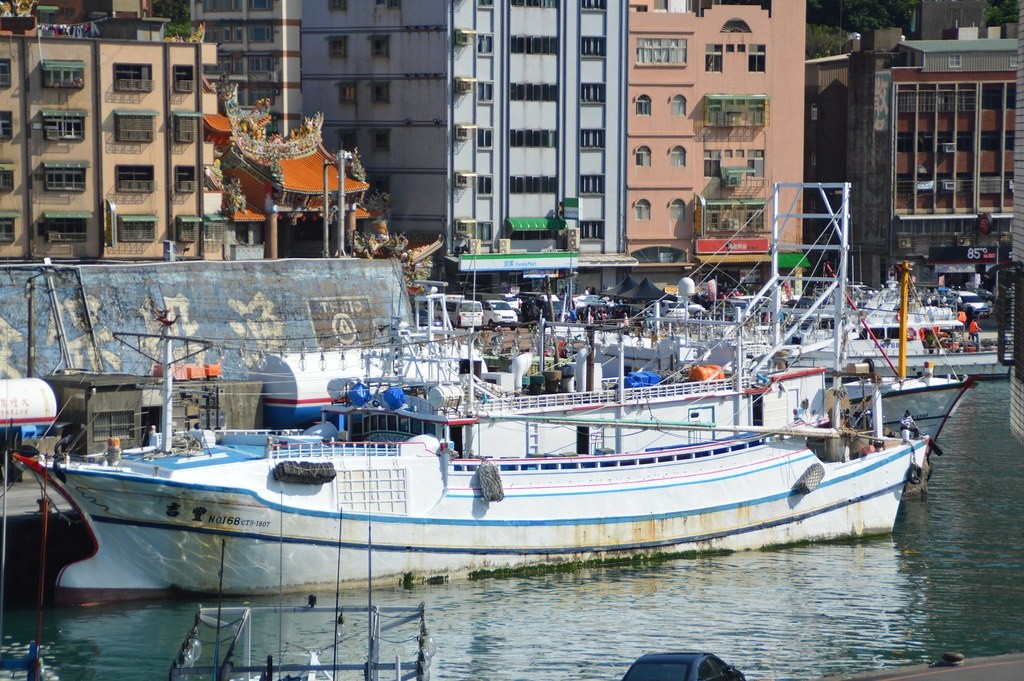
[167,492,436,681]
[788,265,1012,381]
[11,183,980,605]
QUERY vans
[432,300,483,332]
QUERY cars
[951,288,994,316]
[573,295,831,322]
[620,650,746,681]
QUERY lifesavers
[896,311,908,323]
[906,326,917,340]
[907,463,923,485]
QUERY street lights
[992,241,998,292]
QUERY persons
[583,286,598,295]
[956,308,967,334]
[899,411,919,438]
[598,307,607,324]
[968,319,982,343]
[622,312,630,327]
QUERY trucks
[465,293,518,332]
[513,291,570,322]
